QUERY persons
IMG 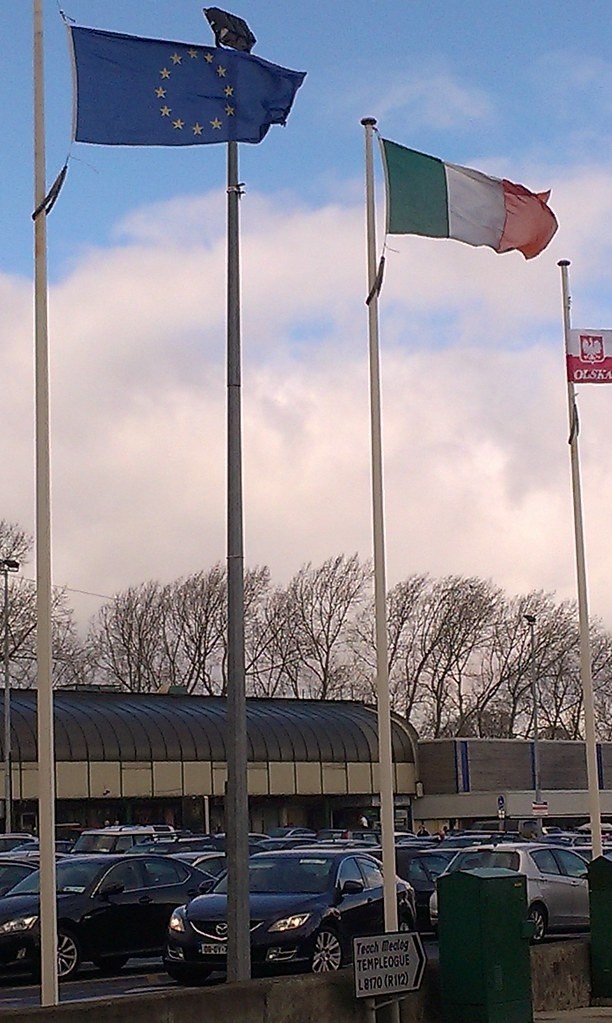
[404,825,450,840]
[359,815,369,828]
[102,821,119,828]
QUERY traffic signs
[352,932,429,1000]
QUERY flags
[381,140,559,261]
[68,26,308,147]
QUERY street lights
[521,613,545,839]
[0,556,23,834]
[198,5,257,984]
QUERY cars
[0,852,221,982]
[430,840,591,943]
[161,845,417,983]
[0,819,612,929]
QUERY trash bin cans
[587,852,611,1000]
[436,868,535,1023]
